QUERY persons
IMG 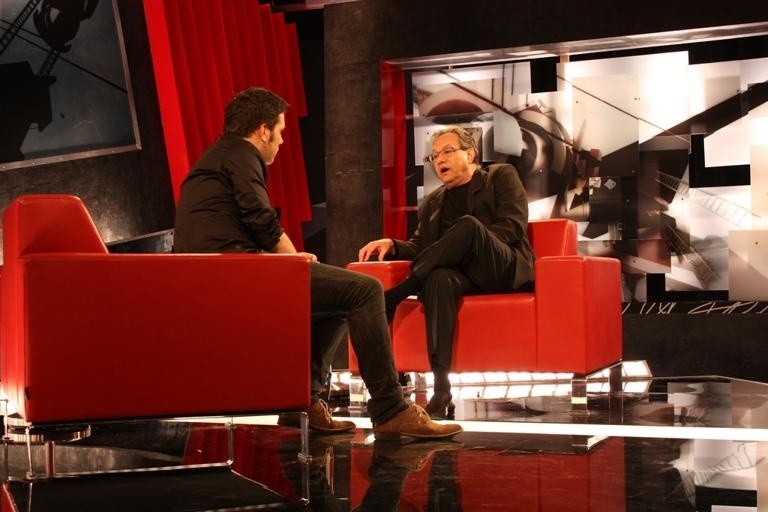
[357,126,539,422]
[168,87,465,438]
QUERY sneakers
[422,383,456,415]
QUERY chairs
[1,192,321,508]
[340,217,626,452]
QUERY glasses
[425,146,462,165]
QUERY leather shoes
[372,405,465,443]
[277,400,356,435]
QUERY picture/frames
[0,0,147,177]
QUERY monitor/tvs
[400,29,768,317]
